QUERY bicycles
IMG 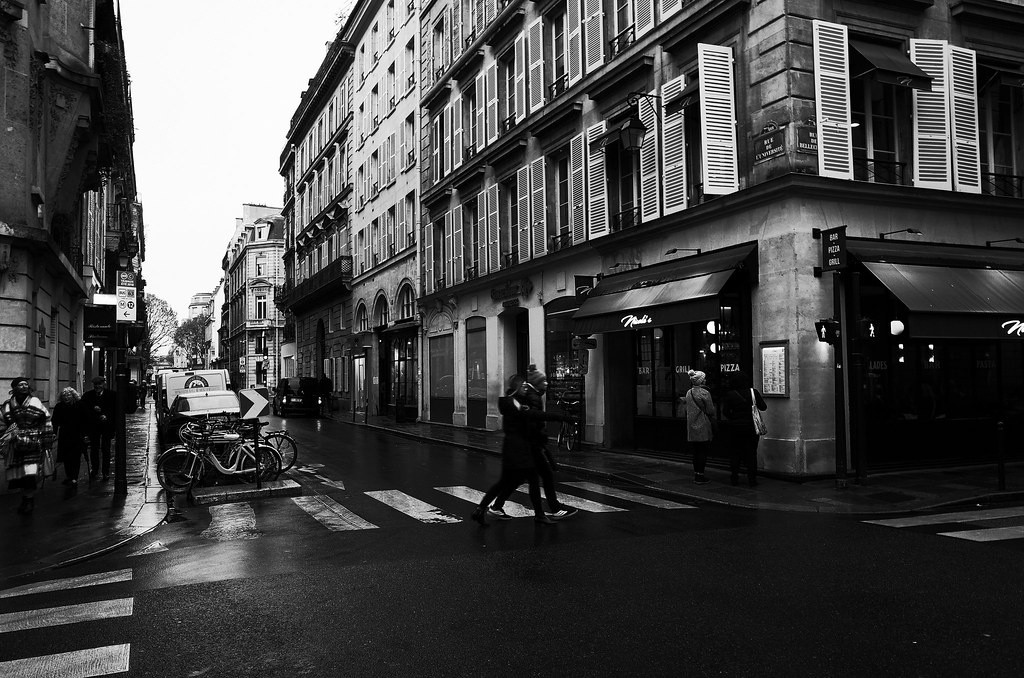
[555,396,580,452]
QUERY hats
[11,377,28,388]
[527,365,546,385]
[688,369,706,385]
[91,376,106,384]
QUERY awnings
[571,239,758,336]
[978,62,1024,93]
[849,39,934,92]
[665,77,700,118]
[861,261,1024,340]
[588,111,638,155]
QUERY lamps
[665,248,701,255]
[986,238,1024,248]
[619,91,662,153]
[608,263,641,269]
[880,228,923,239]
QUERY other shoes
[731,475,738,482]
[63,478,76,485]
[750,482,758,487]
[103,475,110,481]
[19,504,32,513]
[694,474,712,484]
[91,474,98,481]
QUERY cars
[148,368,298,496]
[272,376,326,419]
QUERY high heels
[472,508,490,527]
[534,516,559,527]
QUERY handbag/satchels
[750,387,767,436]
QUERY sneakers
[486,506,513,520]
[551,504,578,519]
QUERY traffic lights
[859,319,881,341]
[815,319,835,344]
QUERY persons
[50,386,85,485]
[0,377,53,433]
[139,380,147,409]
[686,369,717,484]
[721,372,768,486]
[468,368,579,525]
[319,373,336,417]
[82,376,117,480]
